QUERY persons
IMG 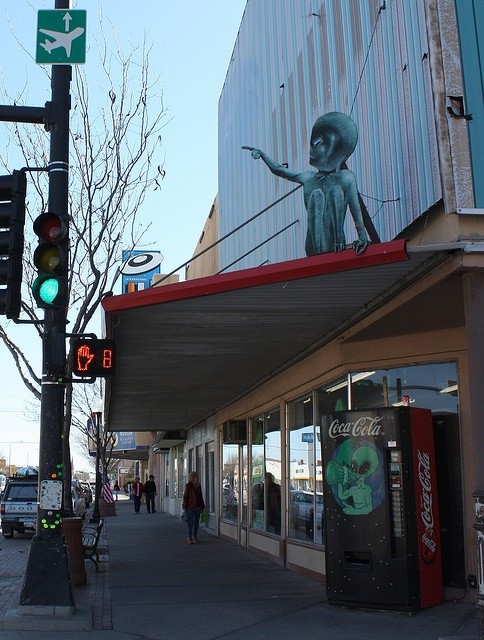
[130,477,142,513]
[142,475,156,513]
[181,472,205,545]
[254,473,280,535]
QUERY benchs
[80,518,104,571]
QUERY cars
[70,487,86,518]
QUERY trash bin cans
[63,516,88,586]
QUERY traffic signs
[35,9,85,64]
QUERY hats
[149,475,155,478]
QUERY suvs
[81,482,92,503]
[71,479,84,495]
[0,472,42,538]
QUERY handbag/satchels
[181,512,189,521]
[200,511,209,523]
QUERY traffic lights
[0,173,27,318]
[32,212,68,311]
[71,340,116,376]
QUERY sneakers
[187,538,192,544]
[192,537,198,543]
